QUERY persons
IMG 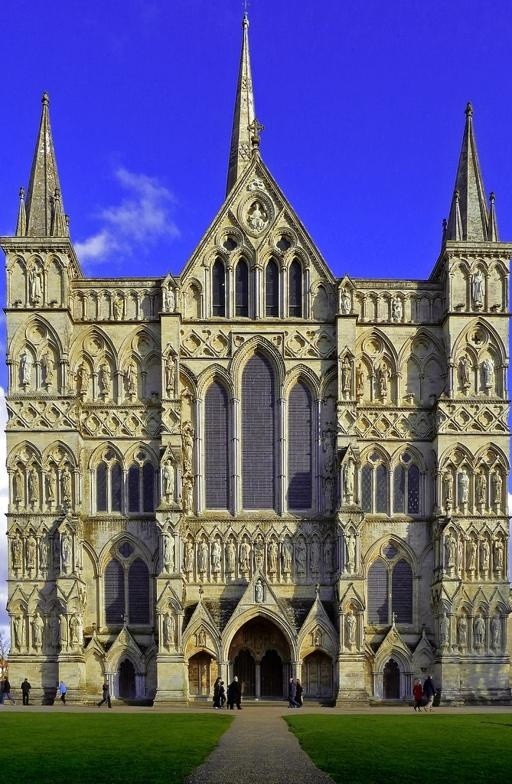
[164,610,176,646]
[57,681,67,704]
[288,678,301,708]
[346,610,356,644]
[21,349,175,395]
[342,288,350,312]
[113,293,124,318]
[346,532,355,565]
[97,680,112,708]
[249,203,266,229]
[13,466,70,502]
[164,460,174,494]
[413,678,423,711]
[345,463,355,505]
[445,468,504,650]
[166,286,175,312]
[3,676,18,705]
[422,675,438,712]
[12,532,75,565]
[473,269,486,301]
[31,265,42,302]
[14,612,86,646]
[20,679,31,705]
[256,580,263,603]
[213,676,243,710]
[342,357,497,389]
[295,679,303,708]
[165,533,175,563]
[393,298,402,321]
